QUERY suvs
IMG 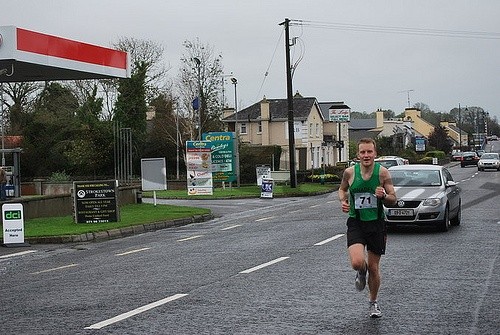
[374,155,409,182]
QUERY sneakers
[369,300,382,318]
[355,260,367,292]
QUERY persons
[339,139,398,318]
[0,167,7,201]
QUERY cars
[382,165,462,232]
[478,153,500,171]
[450,149,488,167]
[487,135,498,141]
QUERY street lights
[193,57,202,141]
[173,102,183,180]
[230,78,238,132]
[458,102,469,152]
